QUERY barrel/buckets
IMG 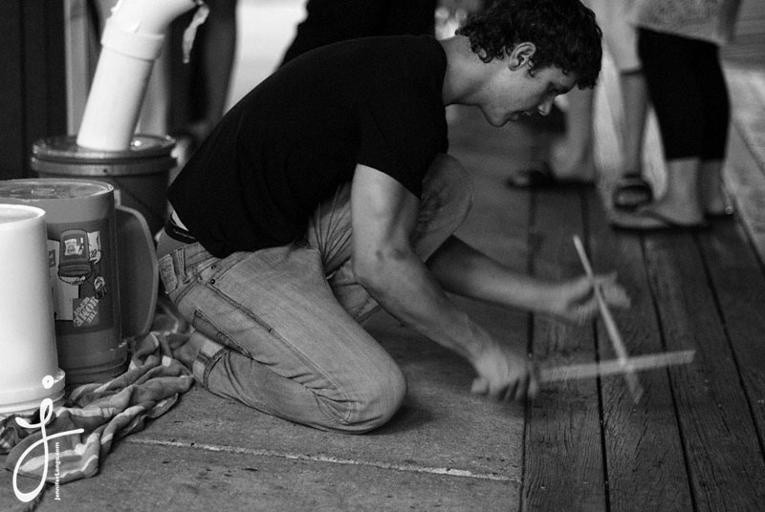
[32,133,179,252]
[0,203,65,420]
[0,179,133,391]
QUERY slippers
[507,158,600,195]
[608,207,707,235]
[612,171,654,212]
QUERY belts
[163,211,198,244]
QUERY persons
[607,0,742,235]
[277,0,440,66]
[155,0,632,434]
[507,0,652,209]
